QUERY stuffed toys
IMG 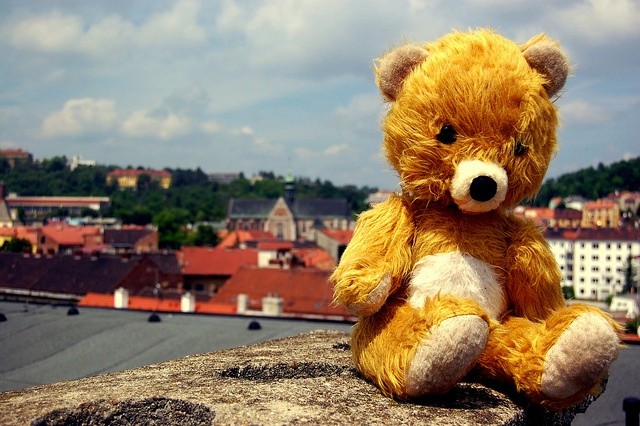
[327,26,628,410]
